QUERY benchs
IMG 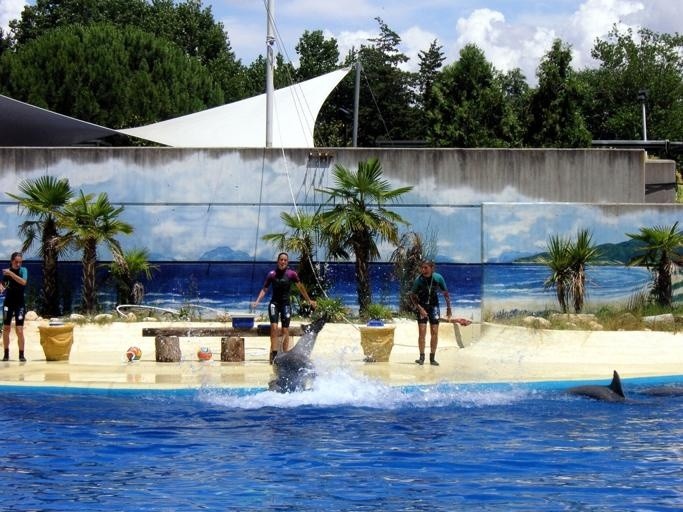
[141,325,306,361]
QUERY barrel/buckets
[121,347,142,361]
[38,323,74,361]
[197,345,211,360]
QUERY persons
[0,252,29,363]
[408,257,453,368]
[250,252,317,368]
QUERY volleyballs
[198,347,212,360]
[126,346,142,361]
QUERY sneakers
[19,358,26,362]
[3,358,8,361]
[430,361,439,365]
[415,360,424,365]
